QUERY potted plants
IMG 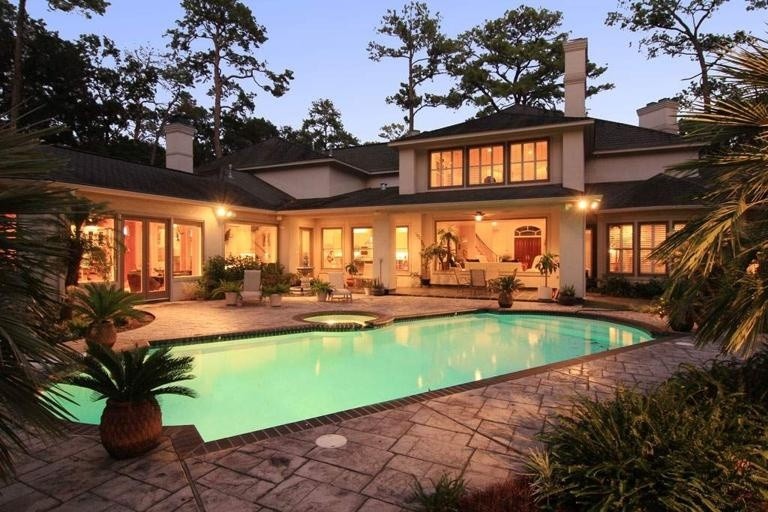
[487,276,526,308]
[261,280,292,307]
[363,275,385,296]
[418,242,436,288]
[56,283,199,461]
[535,252,578,306]
[310,278,336,302]
[209,278,242,306]
[345,262,358,288]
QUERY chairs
[239,269,262,306]
[327,272,352,304]
[453,267,518,295]
